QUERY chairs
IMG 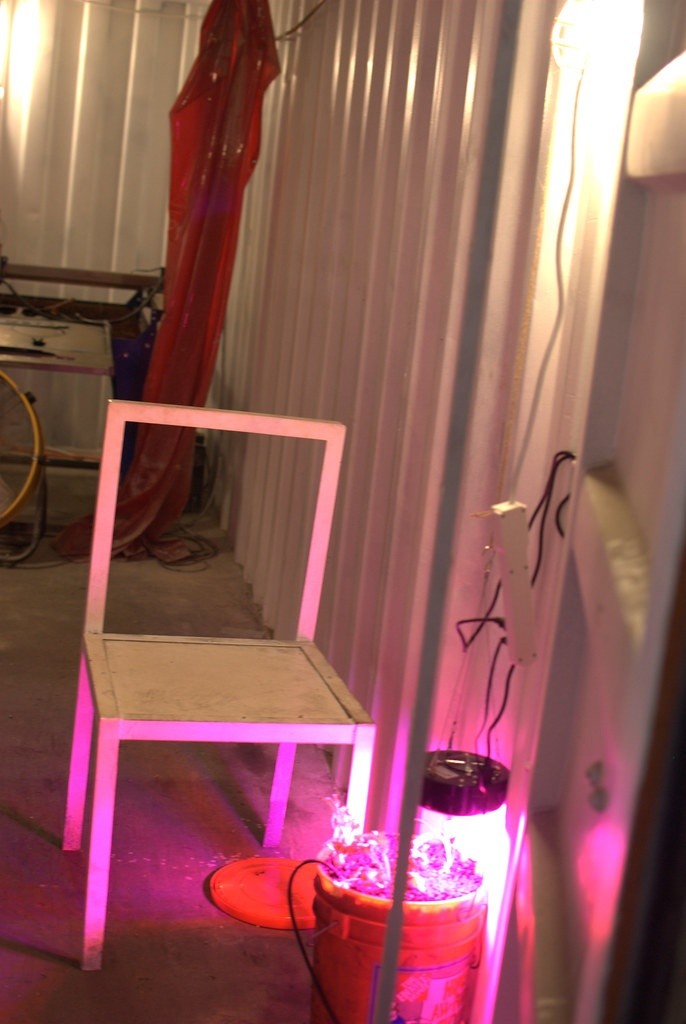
[58,399,381,974]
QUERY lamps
[403,749,516,970]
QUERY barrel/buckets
[414,750,511,1024]
[306,833,485,1024]
